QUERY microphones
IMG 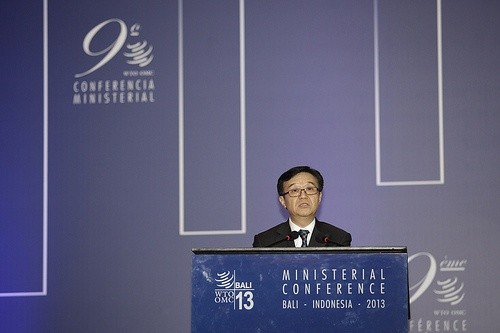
[315,233,344,246]
[265,231,299,246]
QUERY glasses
[280,187,320,196]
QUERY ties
[298,229,309,247]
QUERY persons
[252,166,352,248]
